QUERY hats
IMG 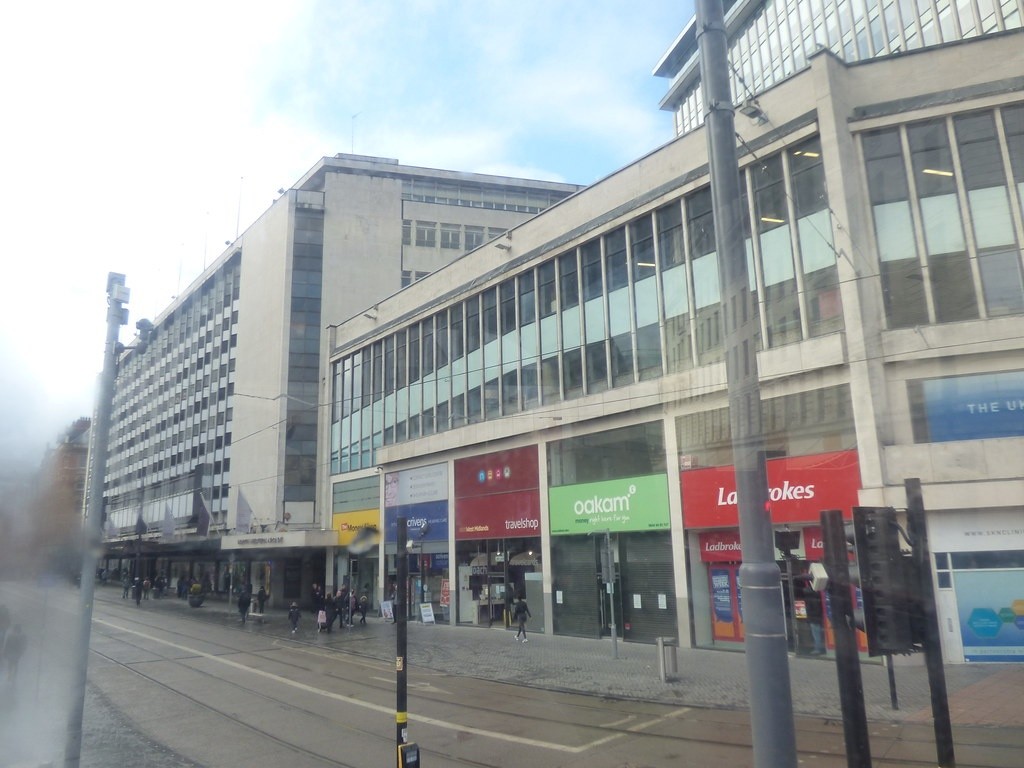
[290,602,298,608]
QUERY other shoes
[522,639,528,643]
[347,624,354,627]
[515,635,519,641]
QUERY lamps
[374,466,383,473]
[363,313,377,321]
[740,105,770,127]
[494,243,513,251]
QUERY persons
[513,595,531,642]
[803,586,826,655]
[101,569,397,632]
[255,586,270,613]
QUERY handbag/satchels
[318,610,326,622]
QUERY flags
[106,490,253,536]
